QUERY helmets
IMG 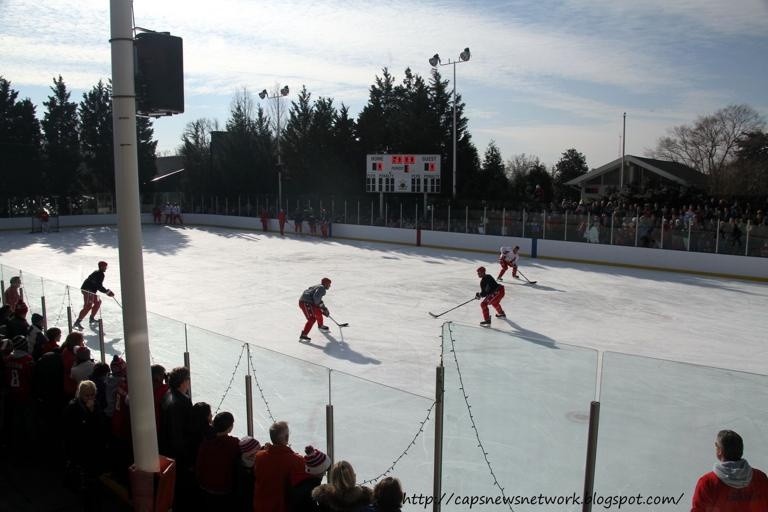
[477,267,486,272]
[98,261,107,267]
[321,278,331,284]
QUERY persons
[690,430,768,512]
[152,202,183,224]
[475,267,505,324]
[293,208,331,240]
[386,199,768,259]
[261,208,269,232]
[40,211,49,233]
[0,276,404,512]
[497,246,519,280]
[277,208,287,236]
[74,262,114,329]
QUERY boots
[89,315,99,323]
[74,321,84,329]
[497,275,503,280]
[300,331,311,340]
[495,311,505,317]
[480,315,491,324]
[318,323,329,330]
[512,272,519,278]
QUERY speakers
[131,33,185,114]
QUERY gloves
[322,307,329,317]
[475,292,481,300]
[106,289,114,297]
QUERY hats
[239,436,261,459]
[12,335,28,352]
[110,355,127,377]
[0,339,13,353]
[31,313,43,324]
[303,446,331,476]
[15,299,28,314]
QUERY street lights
[427,49,472,200]
[258,85,291,218]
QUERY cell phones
[165,373,168,383]
